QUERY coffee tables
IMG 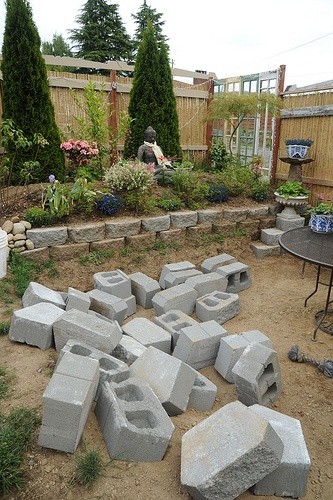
[279,225,333,342]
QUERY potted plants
[285,138,314,159]
[308,204,333,234]
[274,181,310,206]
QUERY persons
[136,126,171,167]
